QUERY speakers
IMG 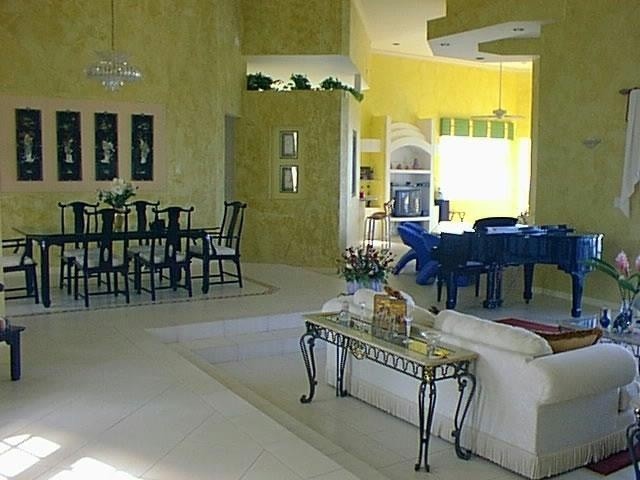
[435,200,449,221]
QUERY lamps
[87,0,143,92]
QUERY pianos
[430,217,604,317]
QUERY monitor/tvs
[391,185,422,217]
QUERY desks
[300,311,479,473]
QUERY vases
[600,309,610,328]
[345,278,384,295]
[621,298,632,326]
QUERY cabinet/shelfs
[387,115,435,245]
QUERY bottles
[413,158,419,169]
[367,185,370,195]
[599,308,611,328]
[360,186,365,201]
[437,187,443,201]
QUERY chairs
[363,199,395,249]
[438,233,480,301]
[0,283,25,380]
[2,201,247,307]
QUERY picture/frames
[279,130,298,159]
[279,165,298,193]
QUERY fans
[471,64,524,119]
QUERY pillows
[535,327,602,353]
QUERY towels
[620,89,640,220]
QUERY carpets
[498,318,640,475]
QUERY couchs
[321,289,640,480]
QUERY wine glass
[339,302,443,361]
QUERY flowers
[97,178,136,208]
[336,245,395,283]
[584,249,640,316]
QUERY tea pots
[360,166,372,180]
